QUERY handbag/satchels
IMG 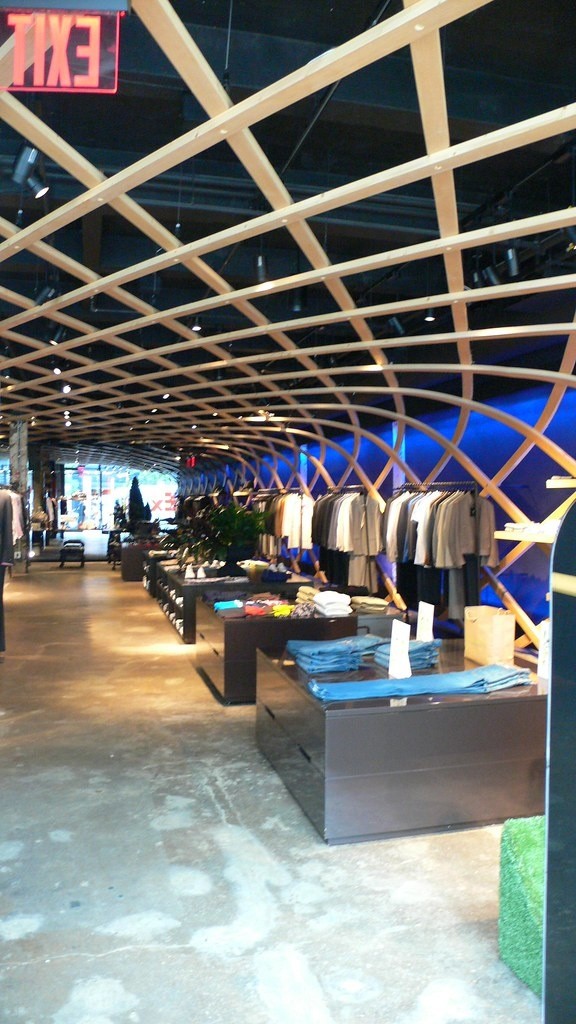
[464,605,516,667]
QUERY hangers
[259,481,478,506]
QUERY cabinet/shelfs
[144,552,356,703]
[257,648,547,846]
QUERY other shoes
[268,564,279,573]
[196,567,207,578]
[184,564,196,579]
[276,563,287,573]
